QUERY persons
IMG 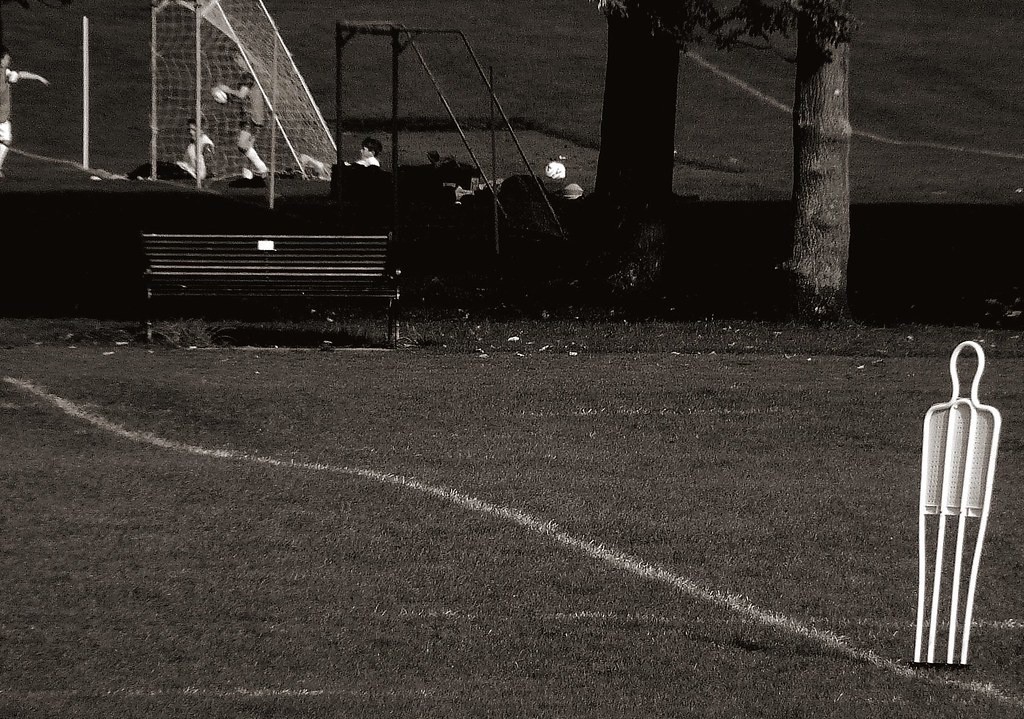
[0,46,50,178]
[213,46,269,183]
[301,138,385,173]
[132,119,215,178]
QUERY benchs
[139,232,403,350]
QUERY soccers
[210,86,230,105]
[545,162,567,179]
[561,183,585,198]
